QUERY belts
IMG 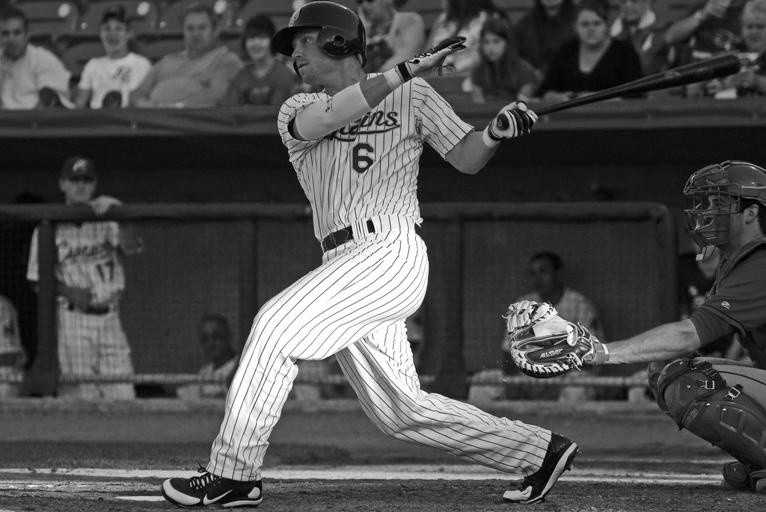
[321,219,424,252]
[69,304,109,315]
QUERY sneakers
[38,83,122,108]
[161,467,264,509]
[502,431,583,504]
[722,460,763,491]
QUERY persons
[468,252,608,401]
[0,295,29,398]
[25,157,146,398]
[0,1,305,110]
[357,1,765,106]
[319,306,425,399]
[161,2,583,508]
[194,315,241,399]
[502,160,766,497]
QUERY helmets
[679,158,766,265]
[270,0,367,77]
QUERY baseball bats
[499,54,741,131]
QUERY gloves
[393,36,468,84]
[487,99,539,143]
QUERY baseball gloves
[505,301,610,377]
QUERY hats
[97,5,131,29]
[58,154,100,179]
